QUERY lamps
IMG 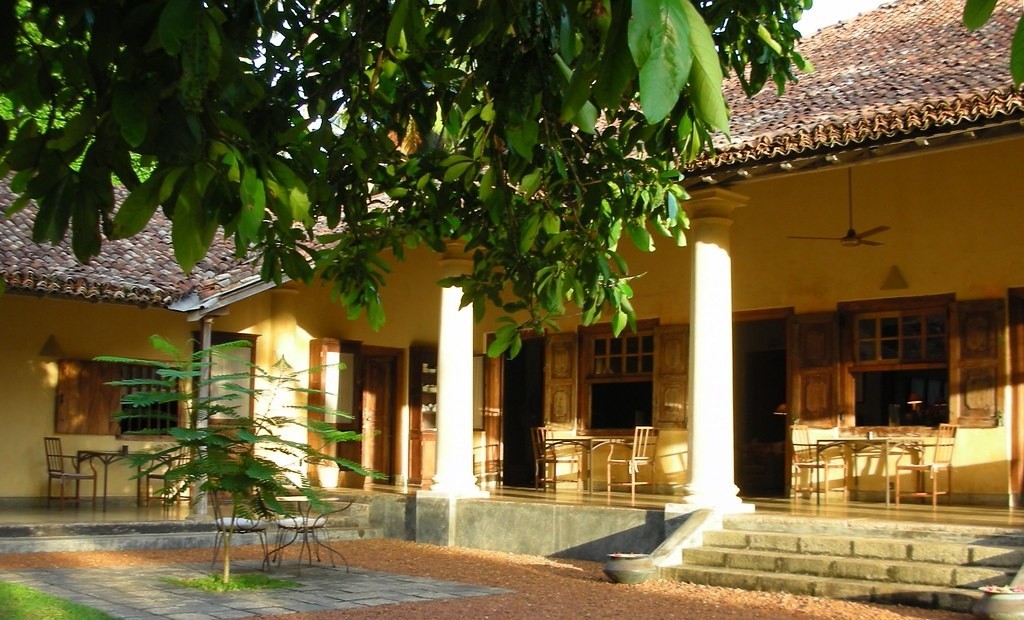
[773,405,787,415]
[906,392,924,412]
[932,397,948,408]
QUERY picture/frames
[856,352,868,404]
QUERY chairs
[895,423,957,512]
[146,447,194,510]
[273,490,337,569]
[529,426,581,493]
[788,423,848,505]
[44,437,97,512]
[607,426,660,501]
[208,489,273,575]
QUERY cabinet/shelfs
[407,345,437,491]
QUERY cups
[123,444,128,454]
[868,432,873,439]
[833,427,839,438]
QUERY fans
[784,166,890,247]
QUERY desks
[542,438,634,498]
[817,436,926,508]
[75,450,171,513]
[222,495,356,574]
